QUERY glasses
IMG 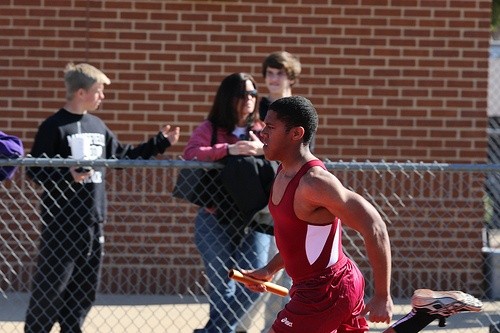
[232,90,258,99]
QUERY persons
[182,72,271,333]
[0,131,24,183]
[240,95,483,332]
[24,63,180,333]
[235,51,315,333]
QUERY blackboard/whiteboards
[482,246,500,301]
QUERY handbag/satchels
[173,160,233,209]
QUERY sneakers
[411,289,484,317]
